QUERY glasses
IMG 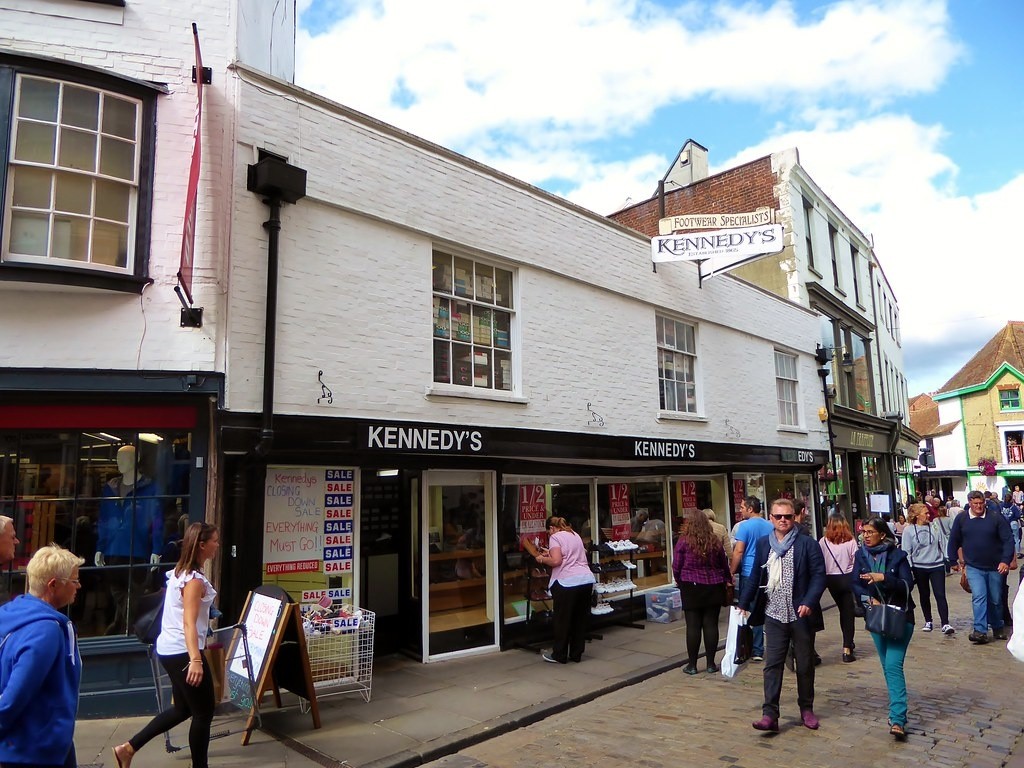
[47,576,80,586]
[770,512,795,520]
[861,529,886,538]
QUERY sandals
[888,718,905,738]
[112,745,132,768]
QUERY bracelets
[541,557,543,563]
[187,660,203,664]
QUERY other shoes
[785,653,794,671]
[752,654,763,661]
[800,710,818,729]
[527,536,640,623]
[542,652,557,662]
[814,657,821,665]
[752,715,778,731]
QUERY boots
[1003,585,1013,626]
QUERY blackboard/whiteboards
[225,584,317,713]
[263,468,325,561]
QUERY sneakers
[969,631,989,643]
[843,653,856,661]
[850,643,855,650]
[922,622,932,632]
[707,665,719,672]
[941,624,954,634]
[683,665,697,675]
[993,627,1007,638]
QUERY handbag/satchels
[720,606,753,679]
[132,589,166,644]
[865,572,909,641]
[724,581,734,606]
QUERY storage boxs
[433,287,511,390]
[434,265,511,389]
[645,586,683,624]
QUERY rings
[1004,568,1008,572]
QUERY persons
[623,508,648,546]
[111,522,220,768]
[849,516,916,736]
[94,445,166,637]
[673,496,860,676]
[634,519,666,576]
[535,516,596,664]
[736,498,827,731]
[0,515,21,566]
[448,493,478,546]
[0,543,85,768]
[882,486,1024,643]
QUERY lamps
[829,346,856,372]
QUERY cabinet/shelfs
[512,536,645,651]
[430,538,663,613]
